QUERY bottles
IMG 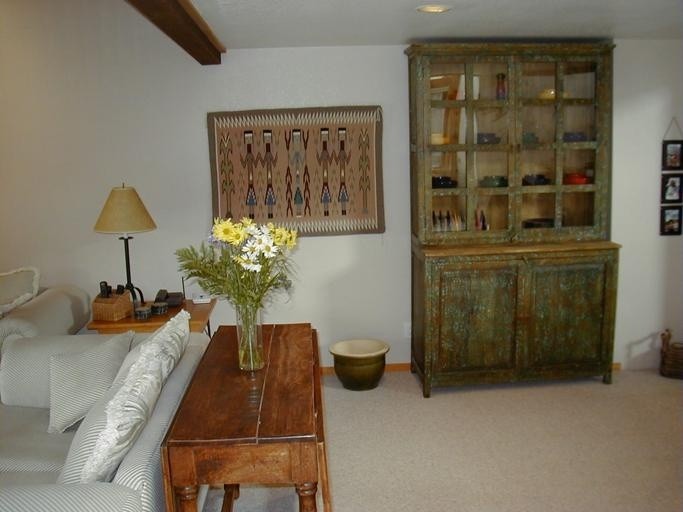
[496,72,505,100]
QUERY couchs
[1,329,211,512]
[0,282,92,358]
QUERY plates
[426,129,588,186]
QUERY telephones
[154,277,185,307]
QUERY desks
[157,324,332,512]
[84,298,216,333]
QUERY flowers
[173,211,301,368]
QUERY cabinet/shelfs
[403,34,618,249]
[410,242,622,399]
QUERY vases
[325,337,391,392]
[235,302,266,372]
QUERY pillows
[41,329,135,437]
[54,308,192,487]
[0,265,42,314]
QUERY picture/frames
[657,205,682,237]
[660,136,683,172]
[659,173,682,205]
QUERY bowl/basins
[522,217,559,228]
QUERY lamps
[92,180,158,311]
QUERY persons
[666,220,674,231]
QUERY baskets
[91,289,132,322]
